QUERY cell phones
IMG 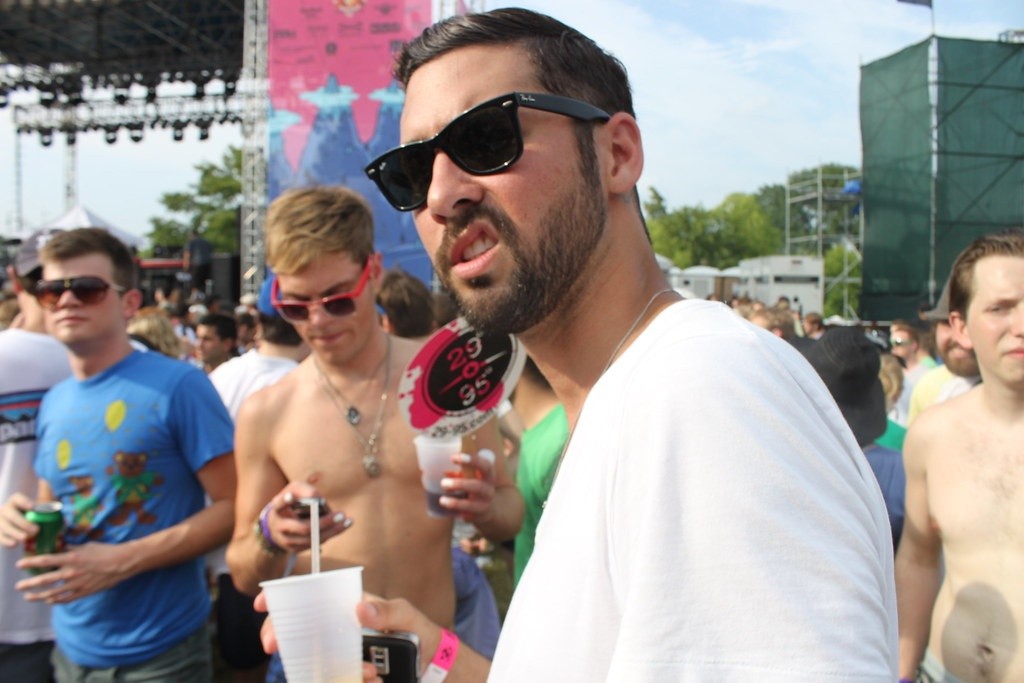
[361,625,423,683]
[289,495,332,519]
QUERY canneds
[23,501,64,575]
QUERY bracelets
[419,626,458,683]
[260,503,277,549]
[253,503,287,558]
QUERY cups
[412,433,464,520]
[257,564,365,683]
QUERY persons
[703,235,1024,683]
[0,187,570,683]
[252,8,901,683]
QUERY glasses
[37,276,129,313]
[271,264,369,322]
[365,91,612,212]
[890,339,915,348]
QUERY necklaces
[319,353,387,424]
[310,331,392,477]
[540,288,675,507]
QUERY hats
[14,227,67,276]
[783,326,887,448]
[259,276,282,316]
[923,274,950,320]
